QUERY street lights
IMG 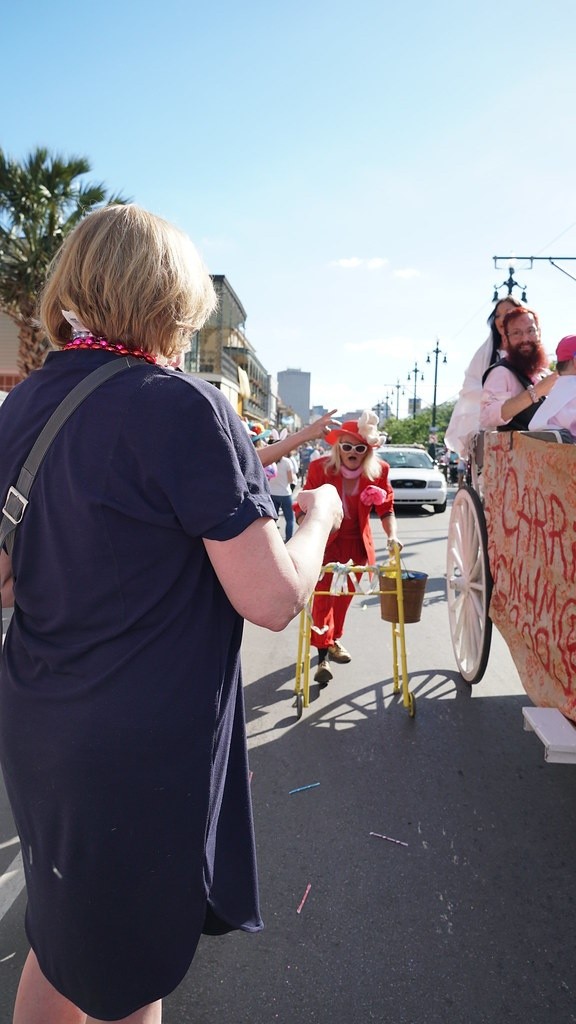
[391,379,406,420]
[373,402,383,417]
[407,361,424,423]
[381,392,392,420]
[426,340,448,433]
[493,267,528,305]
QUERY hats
[250,423,271,441]
[555,335,576,362]
[242,421,257,437]
[325,410,387,449]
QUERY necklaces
[64,330,156,364]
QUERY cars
[369,446,448,514]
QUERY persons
[479,307,557,432]
[1,204,346,1024]
[428,435,466,484]
[242,417,325,544]
[444,295,521,461]
[528,334,576,441]
[292,411,403,683]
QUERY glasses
[337,441,367,454]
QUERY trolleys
[292,539,418,720]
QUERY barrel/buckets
[379,552,428,624]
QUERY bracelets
[527,385,539,403]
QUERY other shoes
[313,661,333,687]
[328,639,352,664]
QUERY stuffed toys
[357,410,387,447]
